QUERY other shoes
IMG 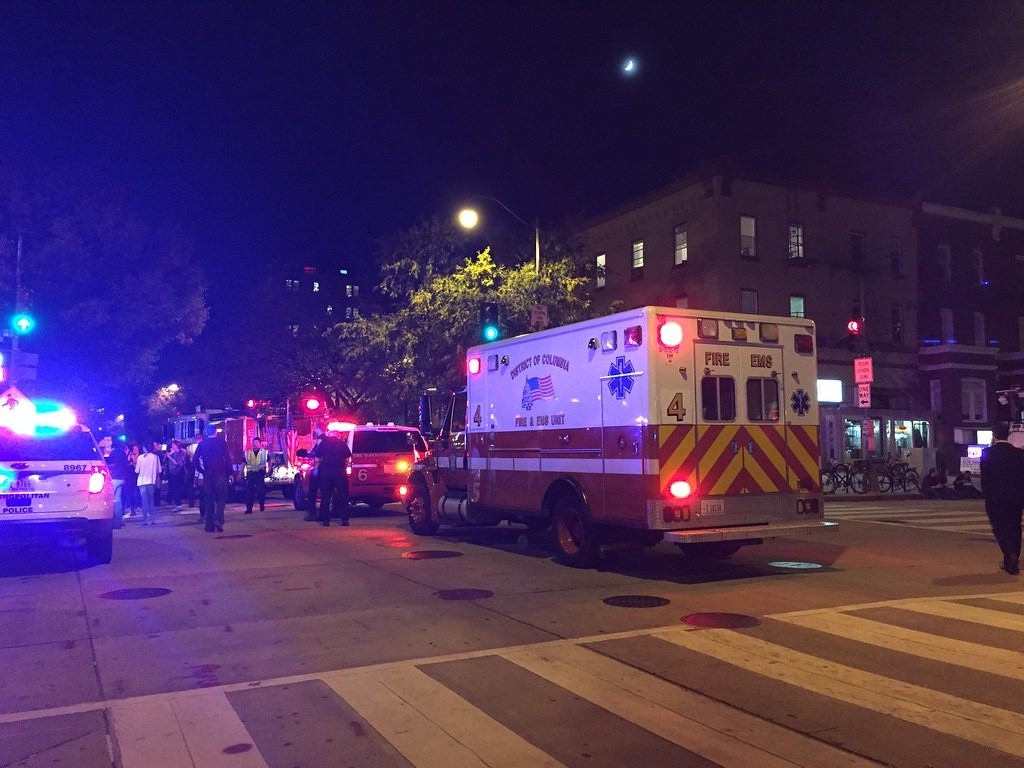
[322,518,329,526]
[129,510,135,515]
[341,518,348,526]
[151,521,155,524]
[999,560,1006,569]
[259,502,265,512]
[215,521,224,532]
[172,506,185,512]
[205,526,214,532]
[245,508,252,513]
[305,512,318,522]
[199,517,205,521]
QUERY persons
[921,466,974,499]
[106,422,353,535]
[980,421,1024,575]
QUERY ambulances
[407,304,830,560]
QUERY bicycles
[819,452,921,492]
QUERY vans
[291,422,432,509]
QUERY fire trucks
[158,400,317,499]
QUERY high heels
[141,520,147,525]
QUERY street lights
[286,392,319,433]
[455,192,543,300]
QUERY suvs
[0,404,115,567]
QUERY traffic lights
[479,301,499,342]
[15,288,35,328]
[849,319,864,334]
